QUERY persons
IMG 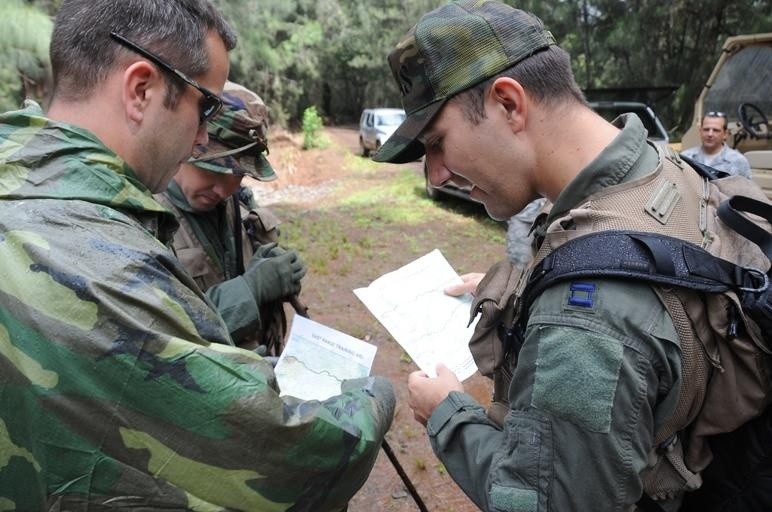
[678,111,752,180]
[150,73,307,366]
[369,0,772,512]
[503,188,552,273]
[0,0,394,512]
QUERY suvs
[676,27,772,205]
[357,107,426,161]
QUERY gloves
[339,372,397,437]
[240,240,309,311]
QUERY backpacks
[518,154,772,512]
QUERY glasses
[108,29,224,126]
[706,110,727,118]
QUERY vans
[417,93,672,215]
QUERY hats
[182,78,279,182]
[371,0,558,166]
